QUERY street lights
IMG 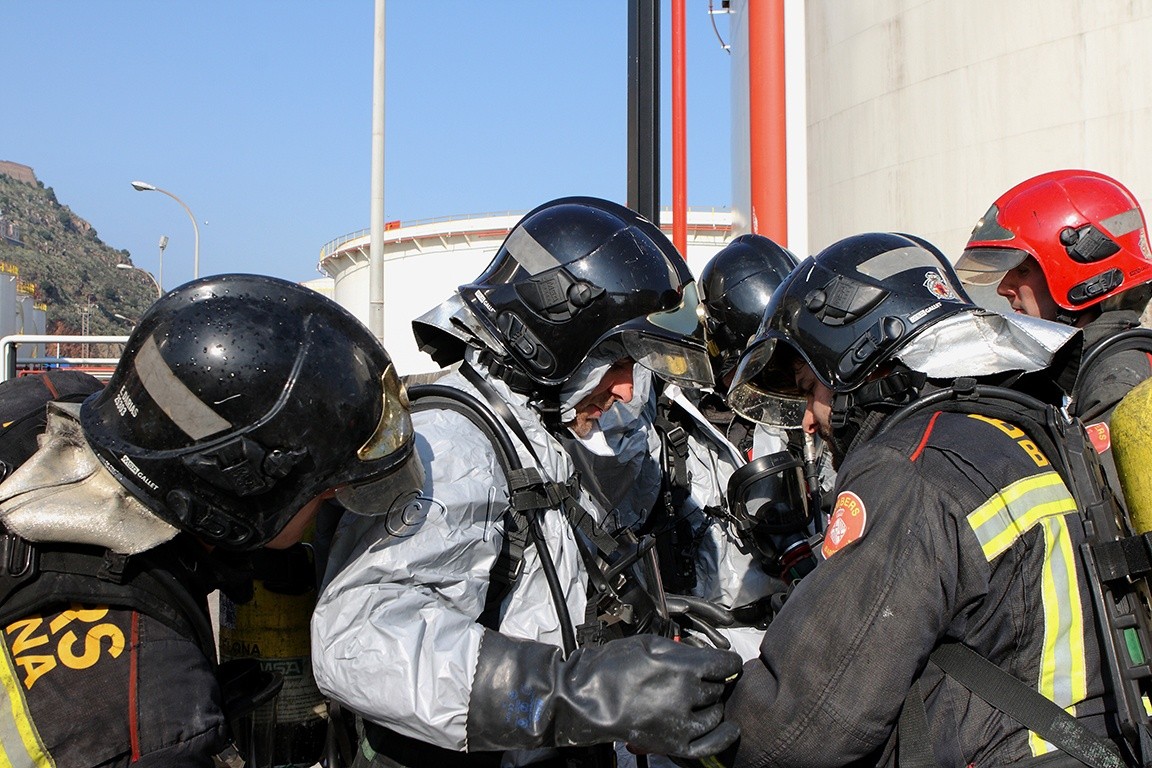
[131,178,199,281]
[117,233,168,299]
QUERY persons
[306,197,804,768]
[957,170,1152,546]
[699,232,1152,768]
[0,274,426,768]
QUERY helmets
[460,195,702,394]
[739,231,984,400]
[78,273,417,553]
[697,233,801,374]
[964,169,1152,314]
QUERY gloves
[466,627,743,761]
[663,591,736,649]
